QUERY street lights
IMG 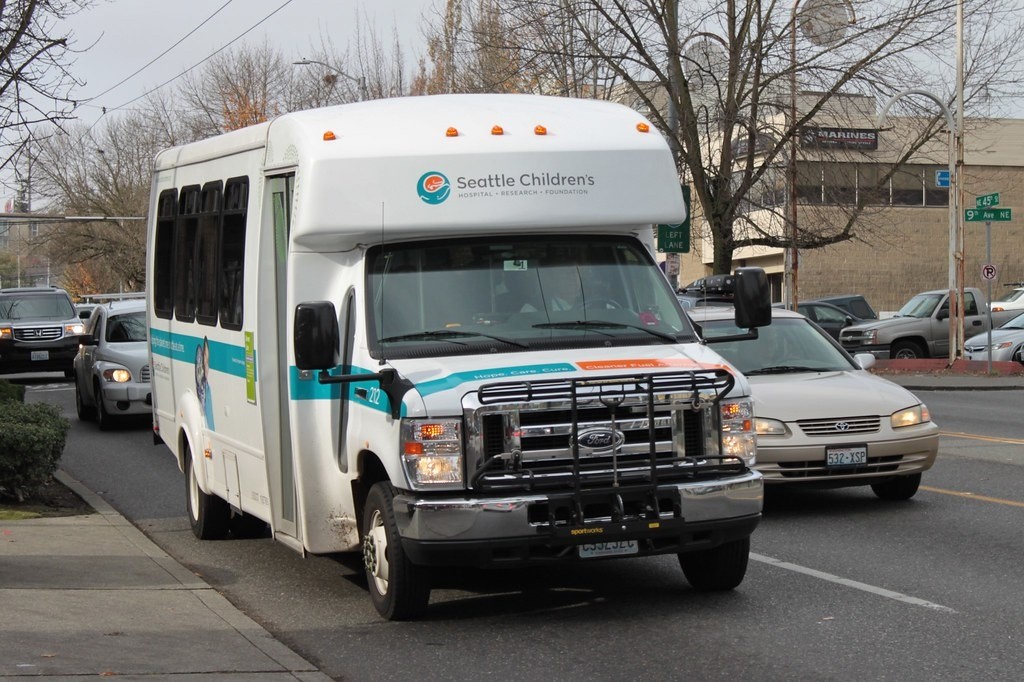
[295,59,366,103]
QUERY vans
[810,295,877,319]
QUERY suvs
[0,285,86,381]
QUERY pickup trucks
[839,287,1024,360]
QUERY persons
[520,259,615,311]
[177,336,215,431]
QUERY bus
[140,92,772,623]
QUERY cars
[683,304,939,517]
[771,301,877,344]
[72,303,101,325]
[71,299,153,432]
[986,288,1024,312]
[675,291,738,307]
[963,312,1024,366]
